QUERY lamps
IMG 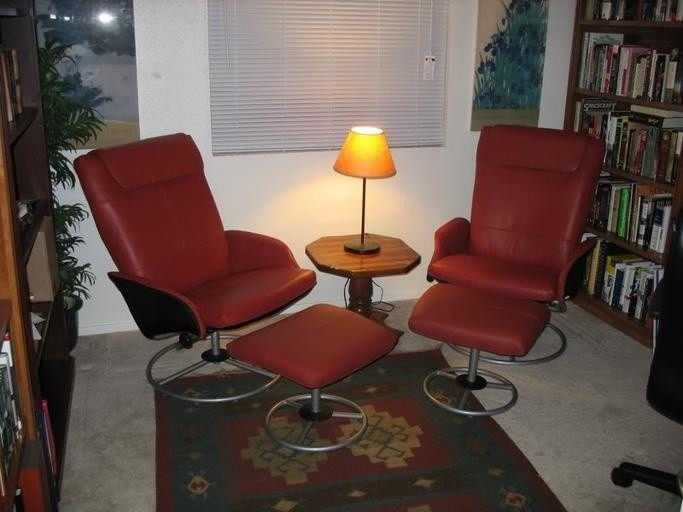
[334,124,397,255]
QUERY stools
[224,305,397,453]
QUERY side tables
[306,233,419,344]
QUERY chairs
[408,124,606,417]
[611,217,682,512]
[73,133,316,402]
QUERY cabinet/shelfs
[563,0,682,349]
[0,0,67,512]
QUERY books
[573,0,683,329]
[0,332,60,511]
[0,47,23,122]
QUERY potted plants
[38,27,104,353]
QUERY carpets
[153,348,570,512]
